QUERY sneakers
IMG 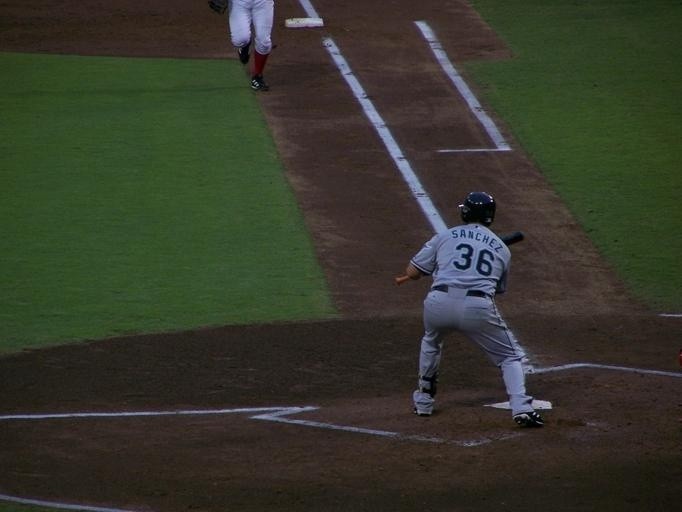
[238,38,252,65]
[248,73,269,91]
[512,411,544,428]
[413,389,432,416]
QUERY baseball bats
[394,232,524,287]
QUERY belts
[431,285,492,298]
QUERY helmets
[457,192,496,227]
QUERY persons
[405,191,545,428]
[227,0,274,92]
